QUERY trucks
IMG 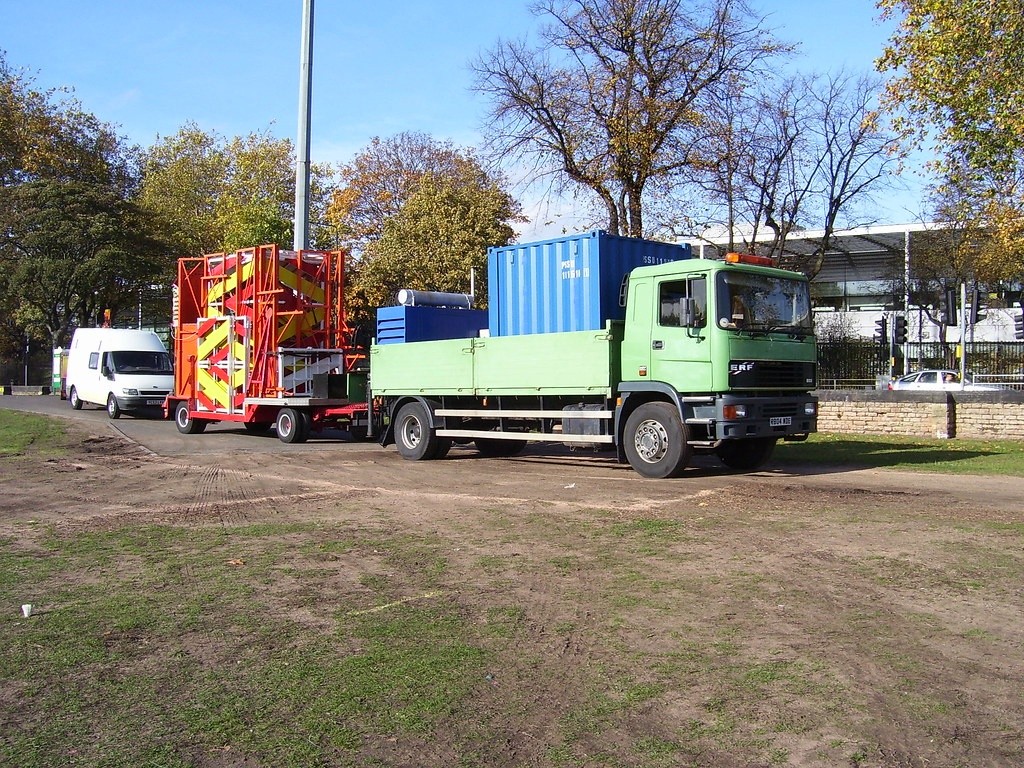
[370,229,819,478]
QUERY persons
[944,374,953,384]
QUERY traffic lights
[1014,315,1024,339]
[970,290,987,323]
[939,286,957,326]
[875,315,887,343]
[895,316,907,344]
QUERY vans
[66,328,174,419]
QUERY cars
[888,370,1016,391]
